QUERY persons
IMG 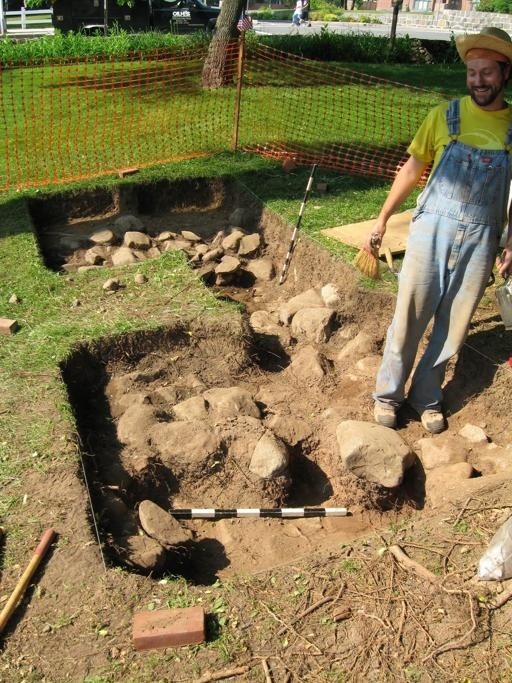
[290,0,311,28]
[296,0,314,28]
[365,21,512,434]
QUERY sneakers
[372,397,398,428]
[410,399,447,434]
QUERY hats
[454,23,512,63]
